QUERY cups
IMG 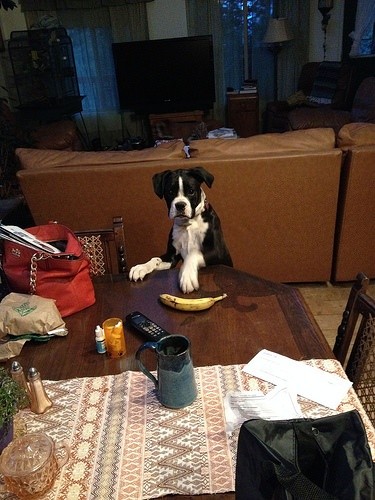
[103,318,127,358]
[136,334,198,409]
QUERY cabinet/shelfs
[149,106,205,145]
[6,26,80,119]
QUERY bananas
[160,293,228,311]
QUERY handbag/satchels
[2,219,96,318]
[234,410,375,500]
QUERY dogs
[128,166,233,294]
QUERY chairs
[50,215,129,275]
[332,272,375,427]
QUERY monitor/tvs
[111,34,216,115]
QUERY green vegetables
[0,368,29,441]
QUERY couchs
[0,57,375,281]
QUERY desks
[0,264,375,500]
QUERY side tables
[227,90,259,137]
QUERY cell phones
[125,311,170,342]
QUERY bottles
[95,325,108,353]
[10,361,33,410]
[27,366,53,415]
[0,431,71,499]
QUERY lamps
[264,17,295,103]
